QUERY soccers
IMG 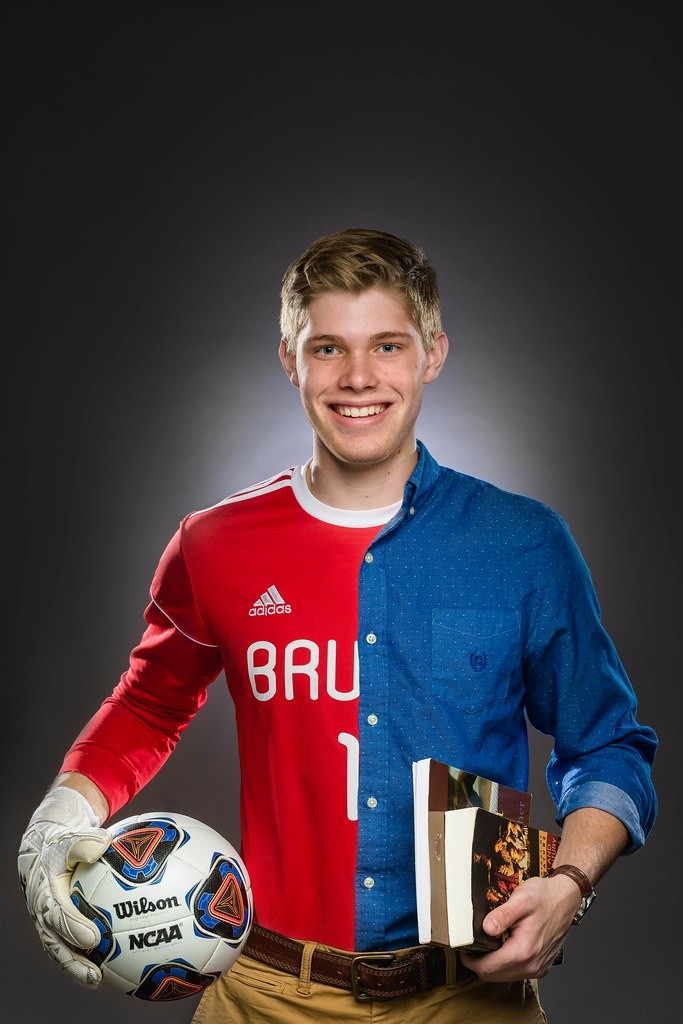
[66,810,253,997]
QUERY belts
[243,924,485,1003]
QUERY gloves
[17,786,109,990]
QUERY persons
[17,225,657,1024]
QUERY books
[412,759,534,952]
[445,807,567,966]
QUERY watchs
[545,865,596,925]
